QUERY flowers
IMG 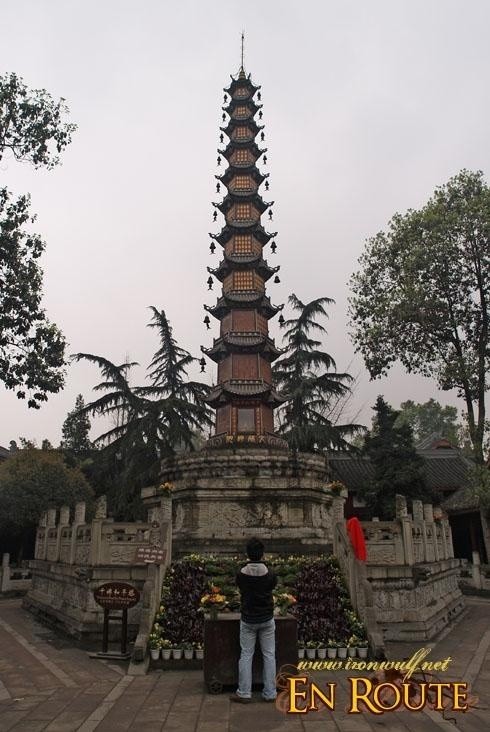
[329,480,344,494]
[150,553,367,646]
[158,483,175,497]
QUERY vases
[151,648,205,662]
[297,648,368,658]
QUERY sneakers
[227,692,278,704]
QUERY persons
[227,534,281,705]
